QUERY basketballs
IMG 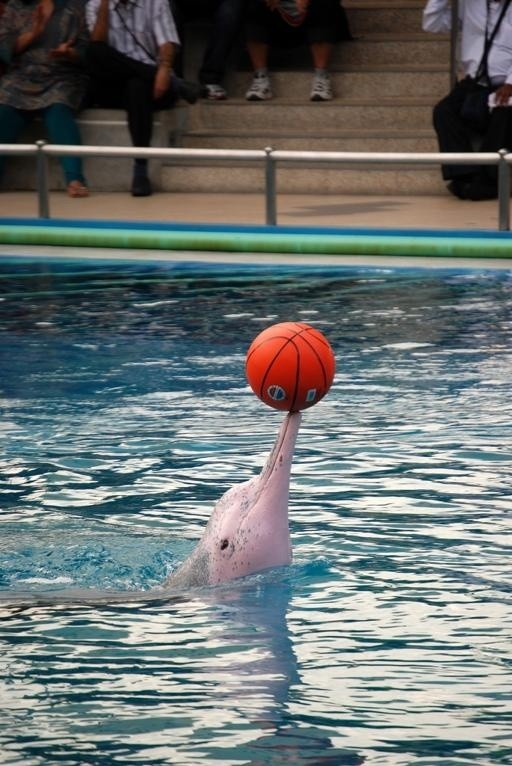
[245,322,335,411]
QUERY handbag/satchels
[172,75,202,105]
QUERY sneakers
[205,82,227,100]
[310,74,333,101]
[68,181,90,197]
[245,70,272,100]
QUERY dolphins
[160,410,302,592]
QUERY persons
[422,2,512,200]
[0,1,351,197]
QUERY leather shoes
[132,173,152,196]
[447,175,496,200]
